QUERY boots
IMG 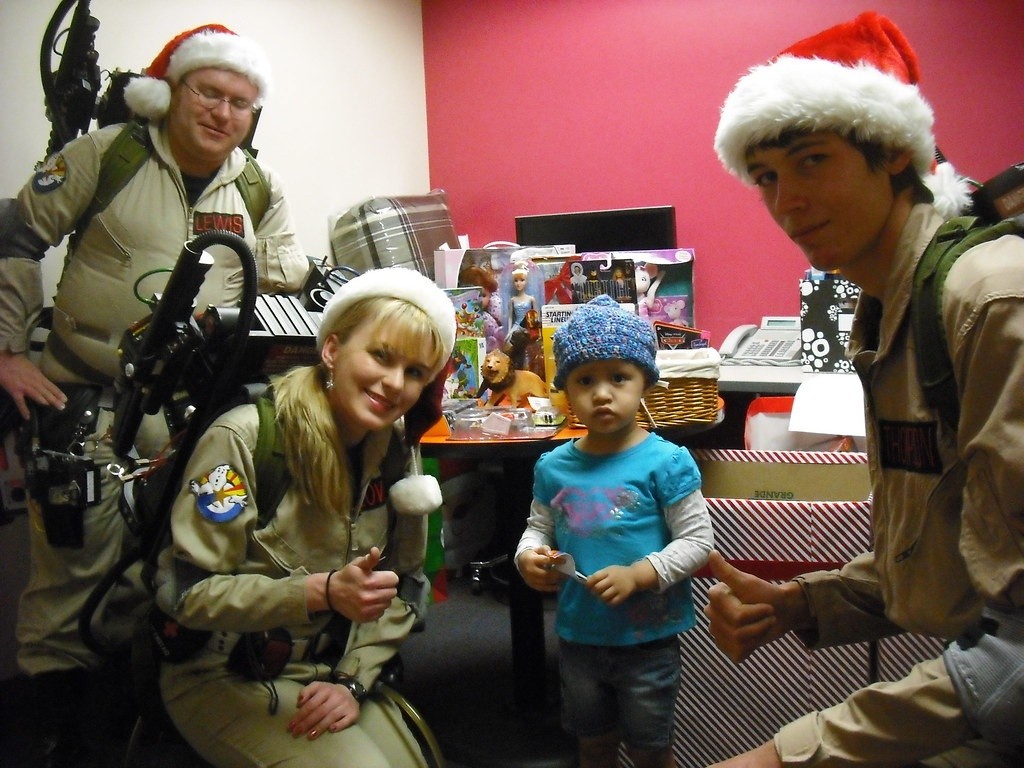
[20,669,84,768]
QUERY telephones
[718,316,802,361]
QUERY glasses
[177,76,256,117]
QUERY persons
[512,293,714,768]
[504,261,536,344]
[704,12,1024,768]
[158,266,457,768]
[0,23,309,768]
[459,266,503,353]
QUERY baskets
[567,345,720,428]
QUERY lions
[481,350,549,409]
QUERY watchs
[335,679,366,701]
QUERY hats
[316,270,455,516]
[122,23,270,119]
[550,294,657,391]
[713,13,974,220]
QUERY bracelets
[326,569,338,611]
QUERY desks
[718,364,808,397]
[416,398,724,716]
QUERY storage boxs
[441,287,487,398]
